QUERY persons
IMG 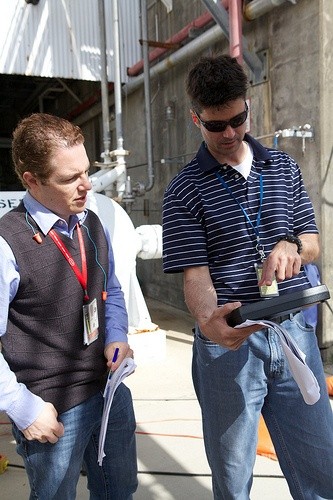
[161,53,333,500]
[0,111,139,500]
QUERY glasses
[195,100,249,133]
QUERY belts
[267,311,300,325]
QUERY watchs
[277,234,303,254]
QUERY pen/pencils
[103,348,120,397]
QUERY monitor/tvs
[225,284,330,328]
